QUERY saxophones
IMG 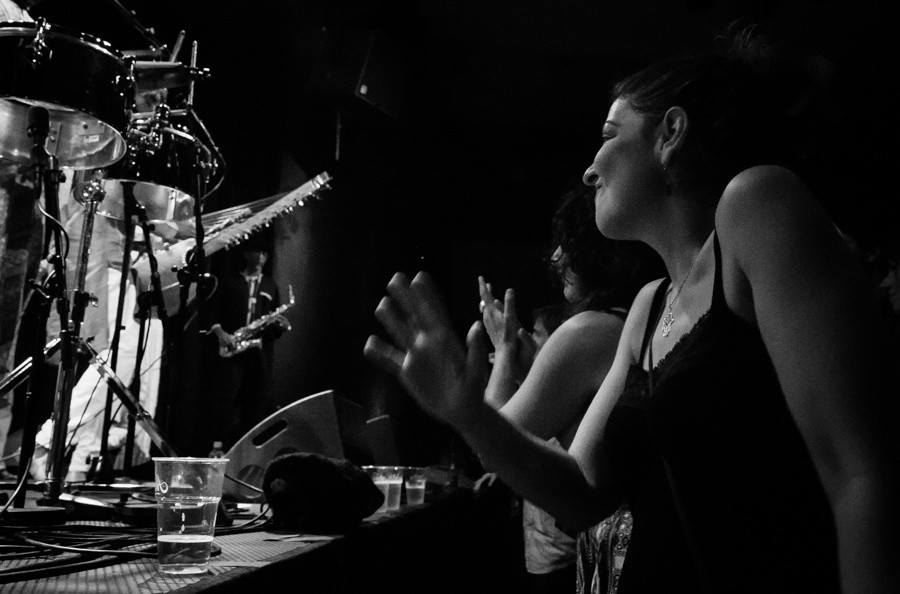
[218,280,304,361]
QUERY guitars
[131,170,338,320]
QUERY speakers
[222,391,397,504]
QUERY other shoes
[17,442,84,484]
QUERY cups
[359,466,429,513]
[152,456,230,574]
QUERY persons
[202,251,294,435]
[364,16,894,594]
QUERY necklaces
[659,258,695,339]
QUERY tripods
[0,160,204,508]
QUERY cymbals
[133,60,215,94]
[118,47,165,60]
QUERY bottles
[209,441,225,459]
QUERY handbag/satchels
[576,507,633,594]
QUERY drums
[68,129,220,224]
[0,18,138,172]
